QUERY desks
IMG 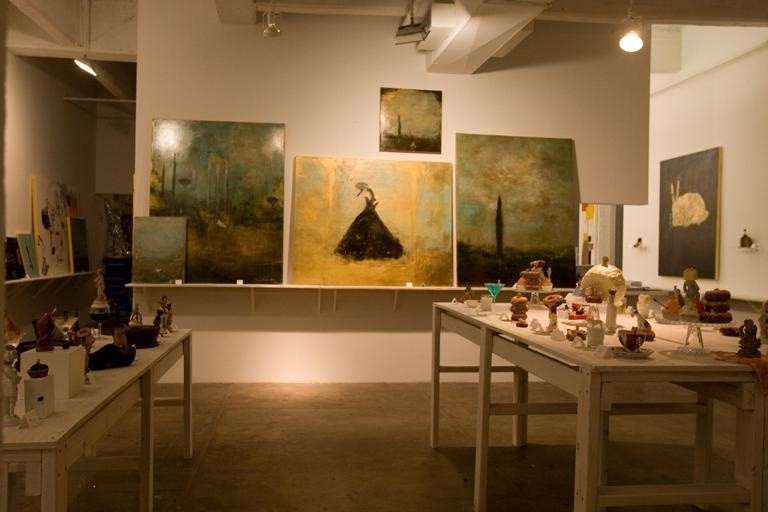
[1,326,199,511]
[427,296,767,512]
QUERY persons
[679,265,706,351]
[333,181,407,261]
[104,200,131,256]
[735,319,763,359]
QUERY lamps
[257,12,283,40]
[394,1,429,48]
[614,1,646,54]
